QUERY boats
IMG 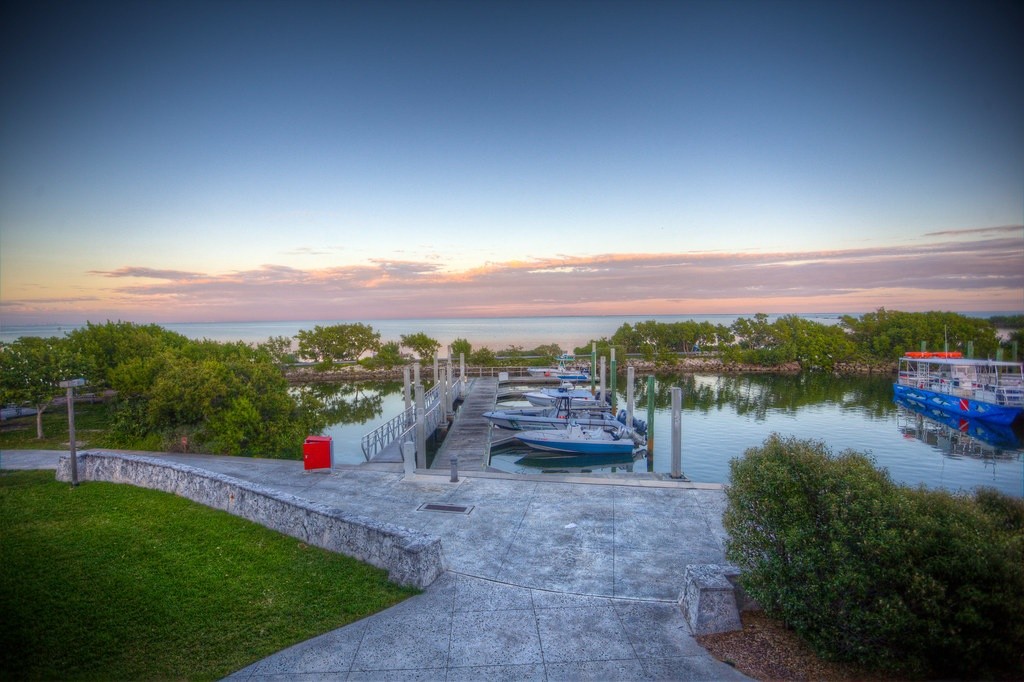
[480,407,650,432]
[894,349,1024,427]
[522,390,612,409]
[893,396,1024,458]
[513,421,650,460]
[528,366,600,384]
